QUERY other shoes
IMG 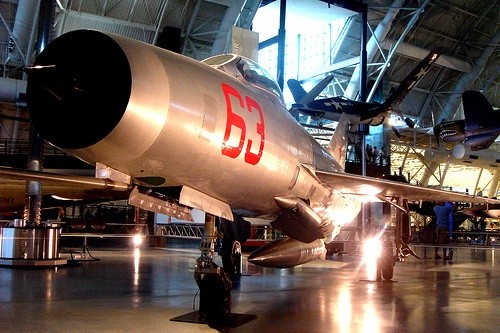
[434,254,442,260]
[443,256,451,261]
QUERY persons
[433,200,457,259]
[433,260,446,265]
[78,203,127,223]
[367,144,386,168]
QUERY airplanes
[390,90,500,161]
[0,26,500,329]
[287,48,443,134]
